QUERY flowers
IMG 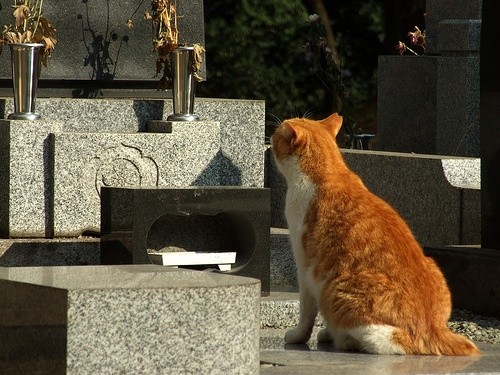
[0,0,58,67]
[127,0,207,83]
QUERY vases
[167,47,200,121]
[7,43,45,120]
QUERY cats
[264,112,482,355]
